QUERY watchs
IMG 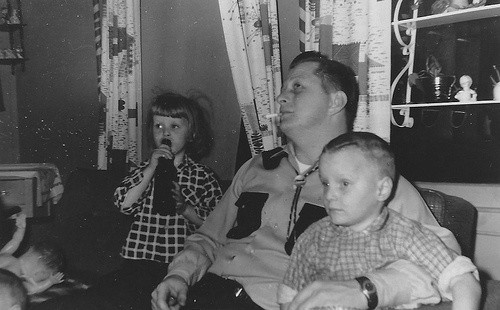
[355,277,379,310]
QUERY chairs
[413,188,500,309]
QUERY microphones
[159,139,171,169]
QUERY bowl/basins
[416,74,456,103]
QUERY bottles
[153,139,177,216]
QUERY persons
[0,204,73,310]
[280,131,483,310]
[111,90,224,310]
[153,51,462,310]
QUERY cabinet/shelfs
[390,0,500,128]
[0,0,28,74]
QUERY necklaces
[287,155,320,240]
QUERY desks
[0,176,54,219]
